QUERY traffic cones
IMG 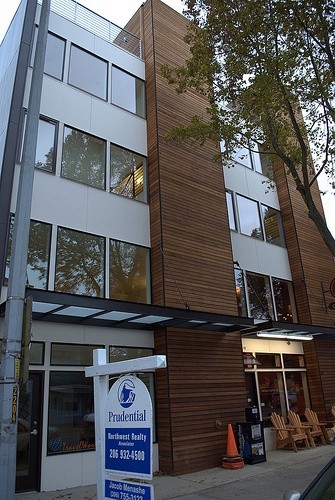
[221,424,244,469]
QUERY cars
[1,419,59,457]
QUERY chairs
[286,409,327,448]
[304,408,335,442]
[269,411,311,453]
